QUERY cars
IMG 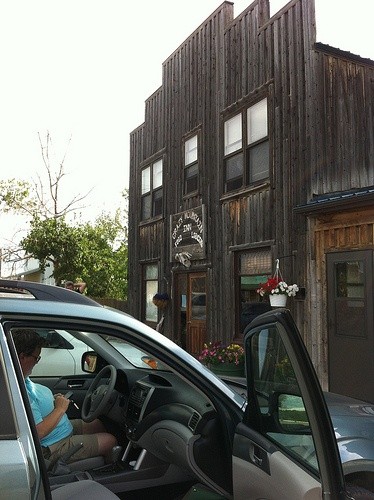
[0,277,374,500]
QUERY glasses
[24,354,41,364]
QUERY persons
[11,328,118,466]
[153,294,168,334]
[64,281,86,294]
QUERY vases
[226,357,237,367]
[269,294,287,307]
[208,362,215,370]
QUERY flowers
[219,342,244,367]
[197,340,220,365]
[257,278,299,301]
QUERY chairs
[51,456,120,500]
